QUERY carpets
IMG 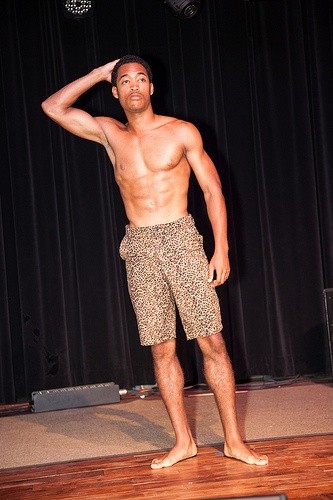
[1,384,333,470]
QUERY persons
[41,54,269,469]
